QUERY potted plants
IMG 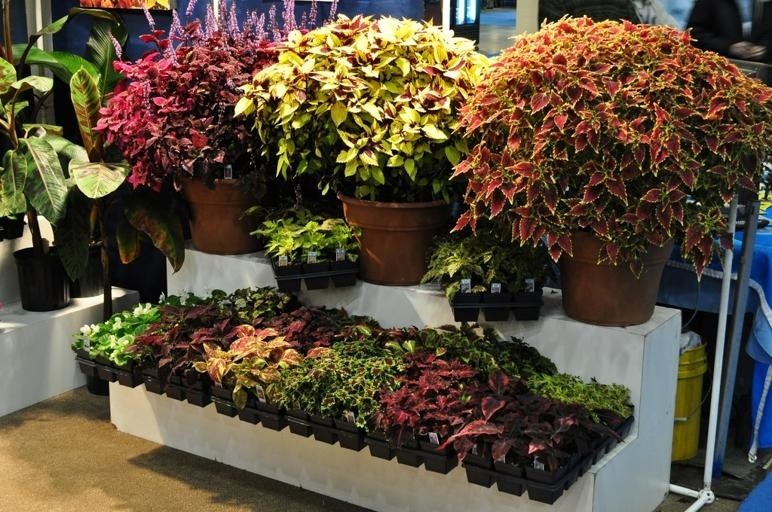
[73,287,636,504]
[0,1,771,324]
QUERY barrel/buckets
[672,343,711,461]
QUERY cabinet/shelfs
[109,236,682,512]
[1,215,140,417]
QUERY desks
[542,178,770,500]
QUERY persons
[538,0,766,60]
